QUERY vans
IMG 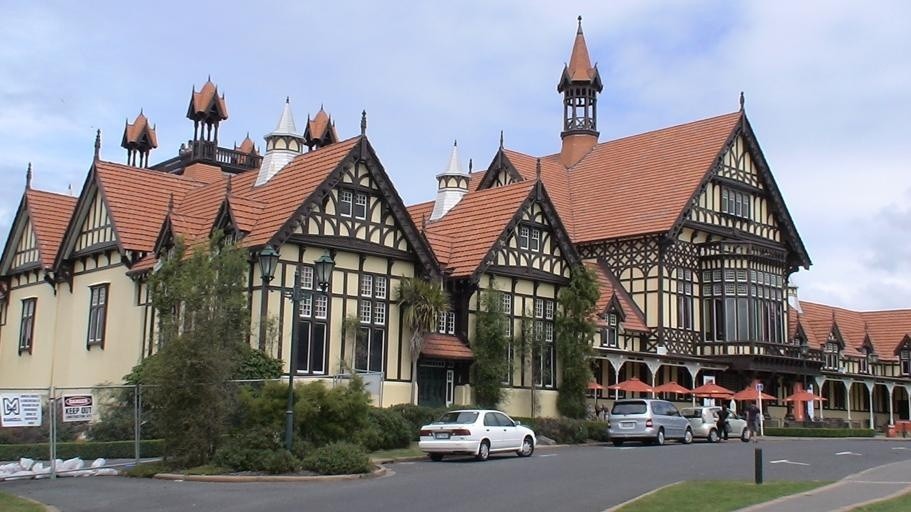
[607,397,698,447]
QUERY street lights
[252,241,338,452]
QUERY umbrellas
[780,390,827,403]
[607,375,654,393]
[587,382,602,390]
[727,386,777,402]
[653,381,689,394]
[689,379,733,399]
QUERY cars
[681,407,753,443]
[418,408,537,461]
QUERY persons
[743,402,759,440]
[712,403,736,444]
[600,401,609,419]
